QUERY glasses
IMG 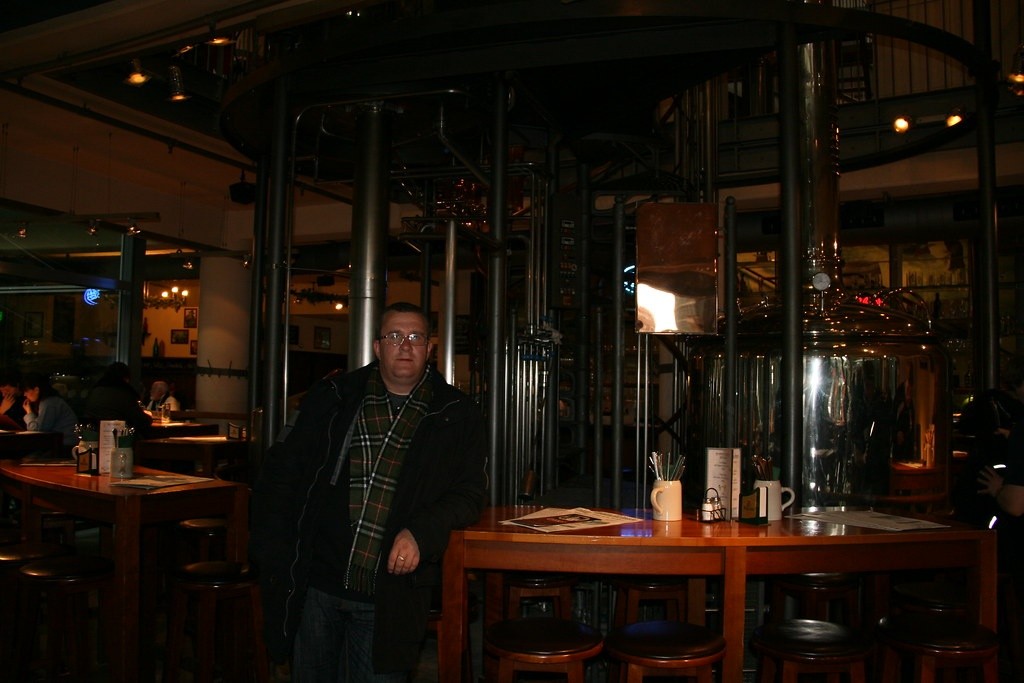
[377,332,429,346]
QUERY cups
[753,480,795,522]
[161,402,170,425]
[71,441,99,474]
[110,447,133,478]
[650,479,682,521]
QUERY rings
[398,556,407,560]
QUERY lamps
[124,57,192,102]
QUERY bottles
[152,337,165,357]
[143,317,148,334]
[702,499,714,521]
[712,497,722,520]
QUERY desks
[0,431,64,456]
[138,436,248,477]
[439,505,999,683]
[0,456,250,683]
[151,423,219,435]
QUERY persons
[815,365,919,498]
[75,360,195,448]
[951,357,1024,607]
[0,365,81,449]
[248,301,491,683]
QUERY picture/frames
[171,308,198,355]
[289,326,299,344]
[314,326,331,350]
[25,311,43,337]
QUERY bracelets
[994,485,1005,497]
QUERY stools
[1,519,270,683]
[482,572,999,683]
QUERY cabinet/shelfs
[902,282,1024,337]
